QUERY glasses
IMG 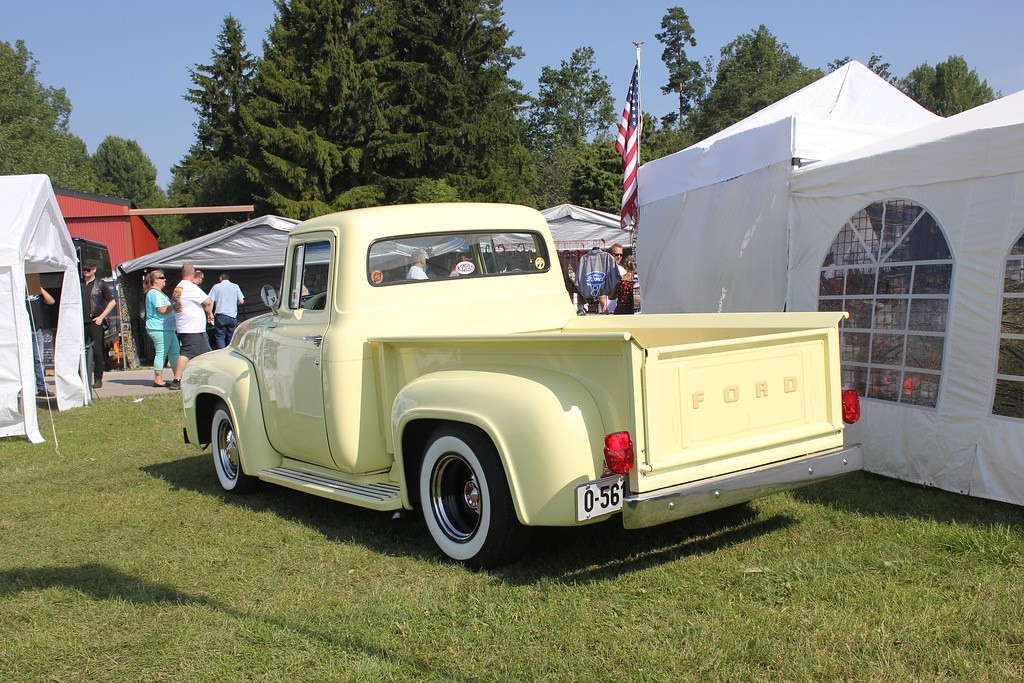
[614,253,623,256]
[156,276,165,279]
[82,269,94,272]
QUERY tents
[430,203,635,258]
[0,173,89,445]
[635,60,1024,506]
[115,203,429,371]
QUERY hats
[82,259,97,270]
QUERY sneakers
[169,380,180,390]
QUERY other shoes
[38,391,54,396]
[93,380,102,388]
[152,381,171,387]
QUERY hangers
[481,238,600,258]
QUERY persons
[142,263,244,390]
[23,287,55,396]
[406,250,428,280]
[80,260,117,389]
[608,255,641,314]
[597,243,627,316]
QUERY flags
[615,62,643,228]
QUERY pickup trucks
[180,202,865,571]
